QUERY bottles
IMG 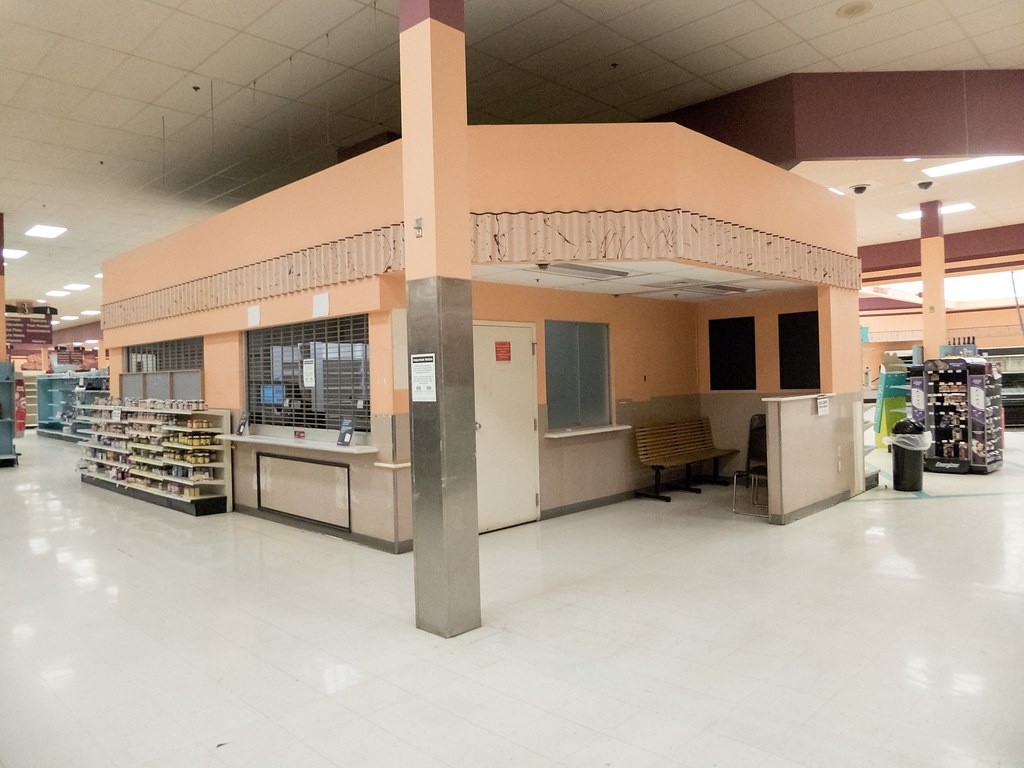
[864,367,871,387]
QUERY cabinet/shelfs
[920,356,1004,476]
[0,360,22,468]
[74,404,234,517]
[24,375,38,429]
[887,346,924,425]
[35,372,110,443]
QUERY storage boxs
[171,465,215,497]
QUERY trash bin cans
[891,418,925,491]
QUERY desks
[215,433,380,454]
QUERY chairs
[732,415,769,518]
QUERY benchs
[633,416,740,503]
